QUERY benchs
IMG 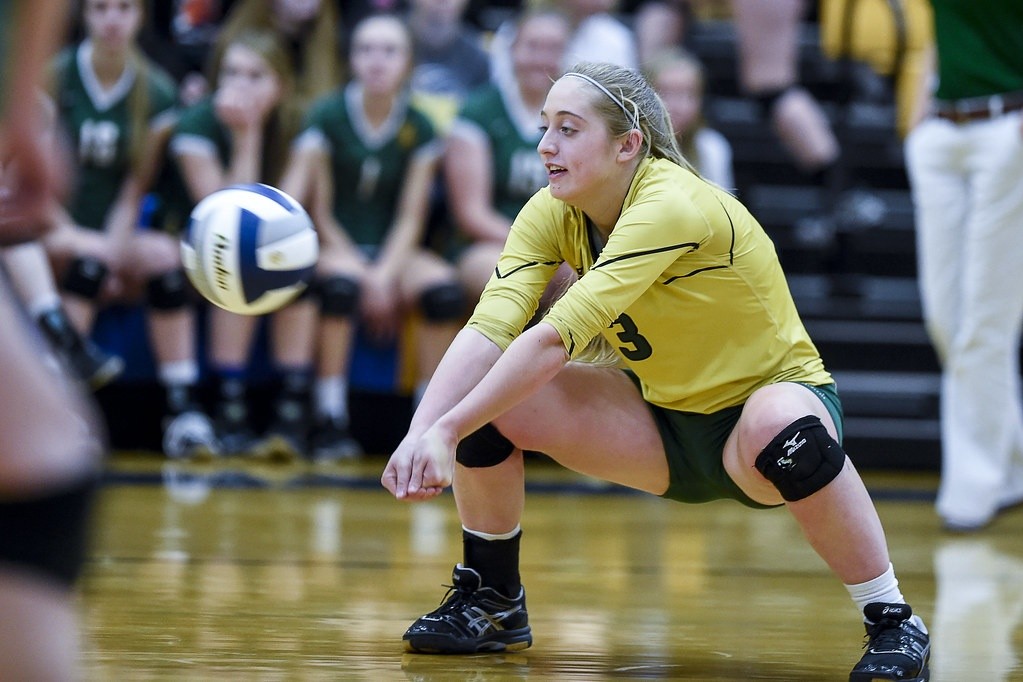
[87,18,944,445]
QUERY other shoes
[163,377,362,467]
[935,480,1022,529]
[39,310,124,389]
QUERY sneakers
[401,651,528,682]
[401,560,533,653]
[848,602,932,682]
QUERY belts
[932,94,1023,123]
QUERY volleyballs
[179,182,319,316]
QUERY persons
[0,276,102,681]
[0,0,1022,528]
[384,65,937,682]
